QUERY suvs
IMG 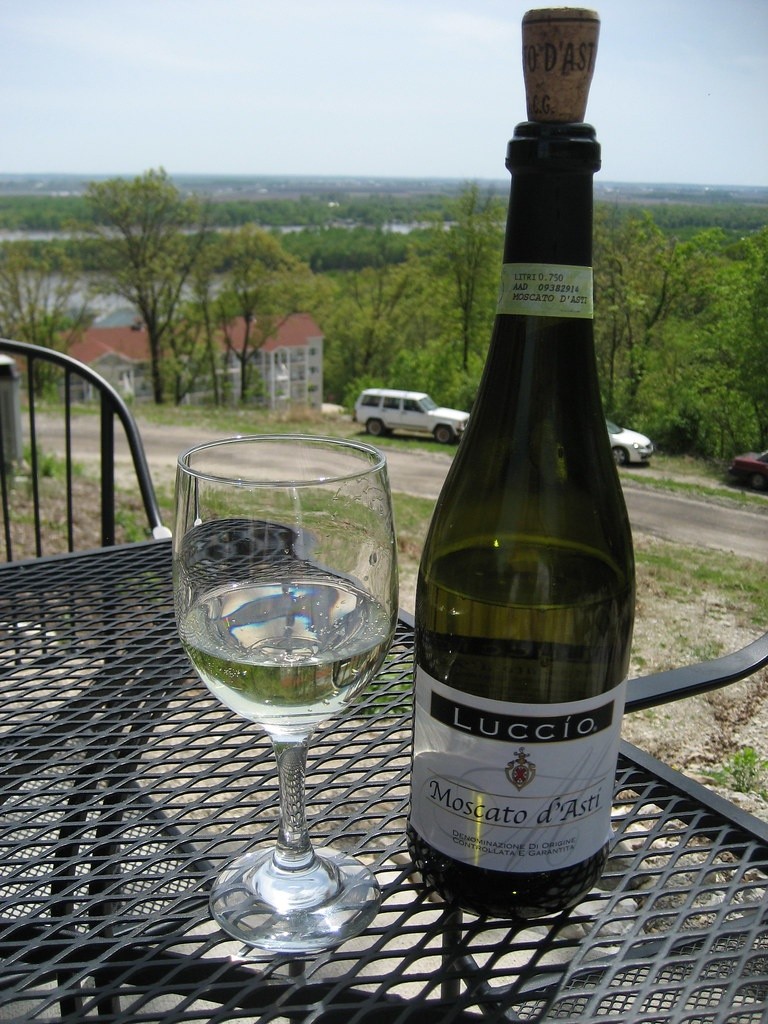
[353,388,471,444]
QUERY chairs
[0,337,218,1024]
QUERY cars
[604,418,655,467]
[728,447,768,492]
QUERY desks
[0,536,768,1024]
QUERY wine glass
[173,435,400,953]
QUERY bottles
[408,120,636,919]
